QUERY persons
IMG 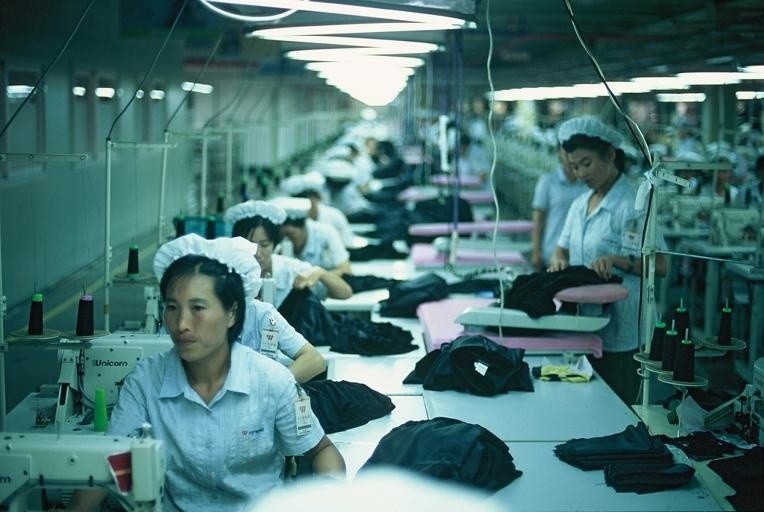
[152,235,329,387]
[224,117,401,307]
[545,116,670,409]
[648,114,763,204]
[422,110,590,271]
[63,230,350,512]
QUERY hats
[226,200,285,226]
[277,145,355,200]
[558,118,622,149]
[273,197,313,219]
[152,233,260,296]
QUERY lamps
[202,0,477,36]
